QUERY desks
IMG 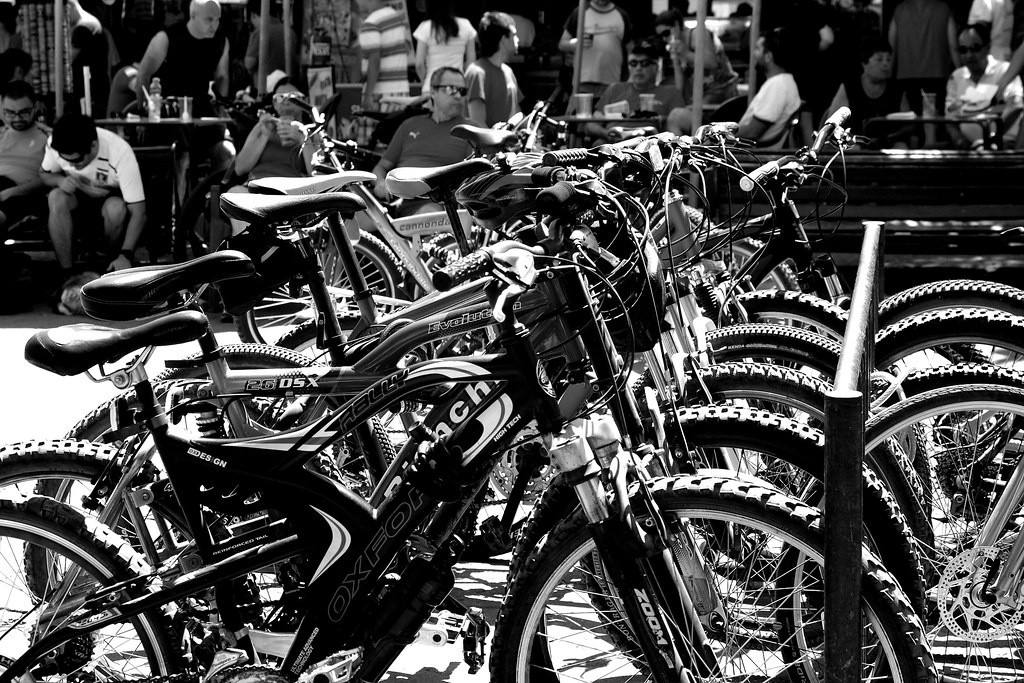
[94,117,235,263]
[869,113,1005,152]
[553,114,667,147]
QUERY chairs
[713,100,808,222]
[311,91,345,131]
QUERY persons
[37,110,147,301]
[370,67,491,220]
[356,0,410,157]
[459,12,527,128]
[134,0,235,263]
[558,0,1024,156]
[412,0,478,99]
[0,79,48,230]
[225,74,326,237]
[0,1,300,120]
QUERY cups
[276,116,295,146]
[639,93,654,111]
[178,98,193,123]
[923,93,936,119]
[148,96,162,123]
[574,94,592,119]
[603,100,630,115]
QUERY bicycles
[1,92,1024,683]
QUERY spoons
[182,96,189,119]
[142,85,154,109]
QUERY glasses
[3,106,33,117]
[954,43,985,54]
[272,92,304,103]
[433,84,468,96]
[628,59,657,67]
[656,26,671,40]
[58,152,85,163]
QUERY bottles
[149,77,161,96]
